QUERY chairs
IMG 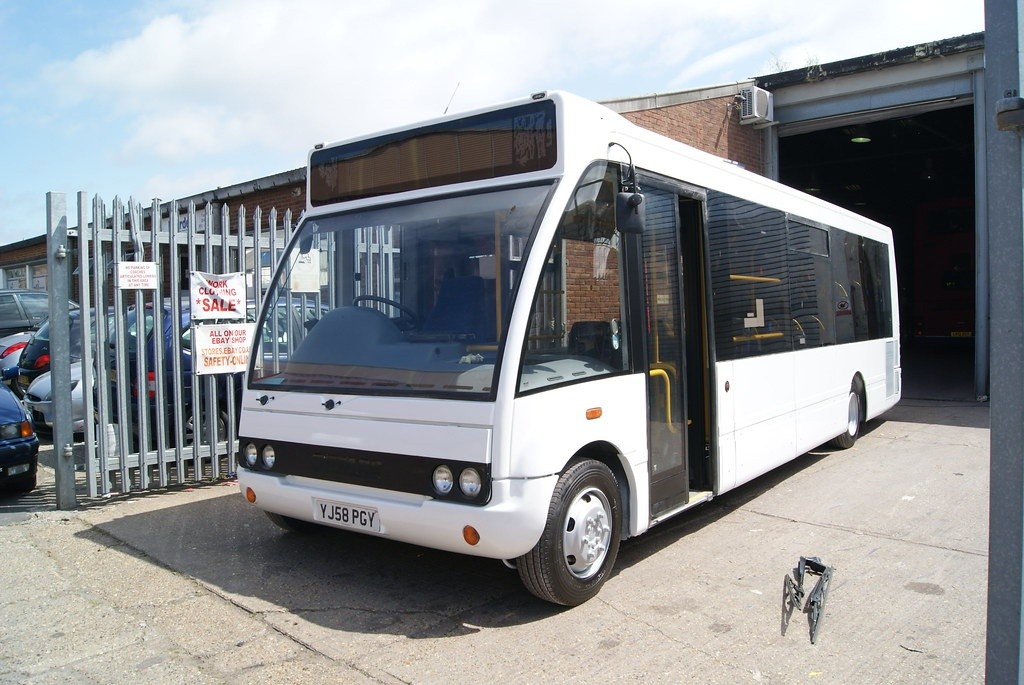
[569,321,613,362]
[423,276,486,339]
[750,280,867,351]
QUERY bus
[232,86,903,609]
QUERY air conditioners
[740,86,773,124]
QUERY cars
[0,287,338,505]
